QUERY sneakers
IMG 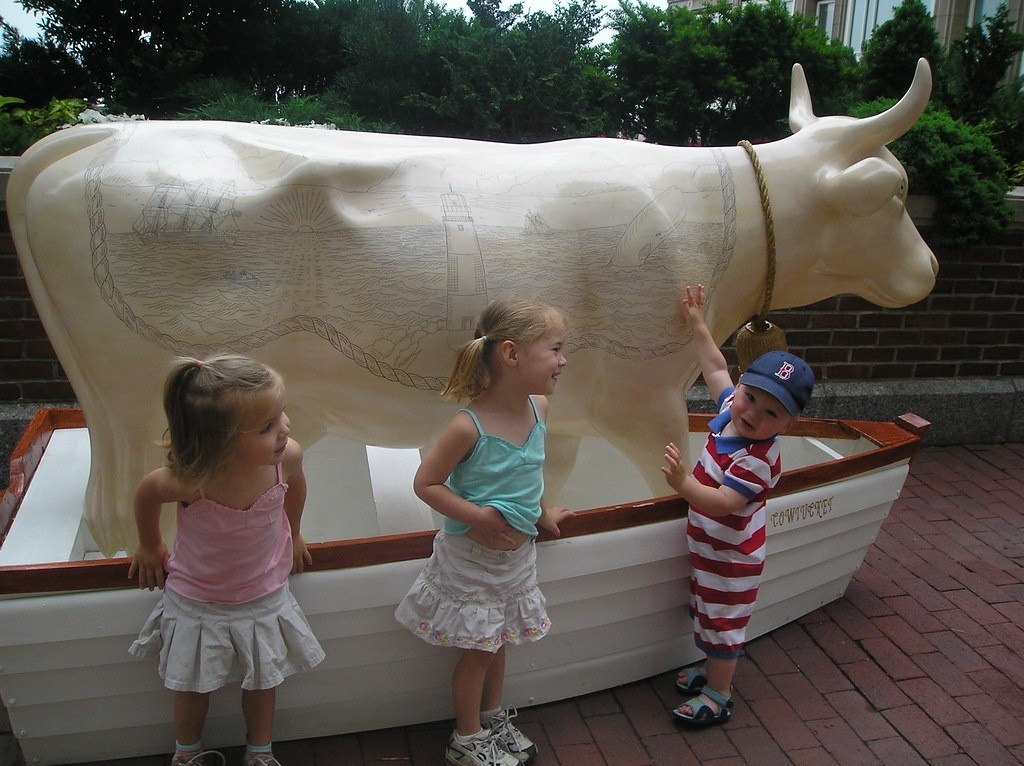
[479,709,540,762]
[441,729,523,765]
[172,748,211,764]
[243,745,286,765]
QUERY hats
[738,349,815,418]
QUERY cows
[5,56,939,560]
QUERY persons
[390,295,571,766]
[123,352,315,766]
[655,283,817,730]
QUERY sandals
[668,660,711,689]
[673,687,735,728]
